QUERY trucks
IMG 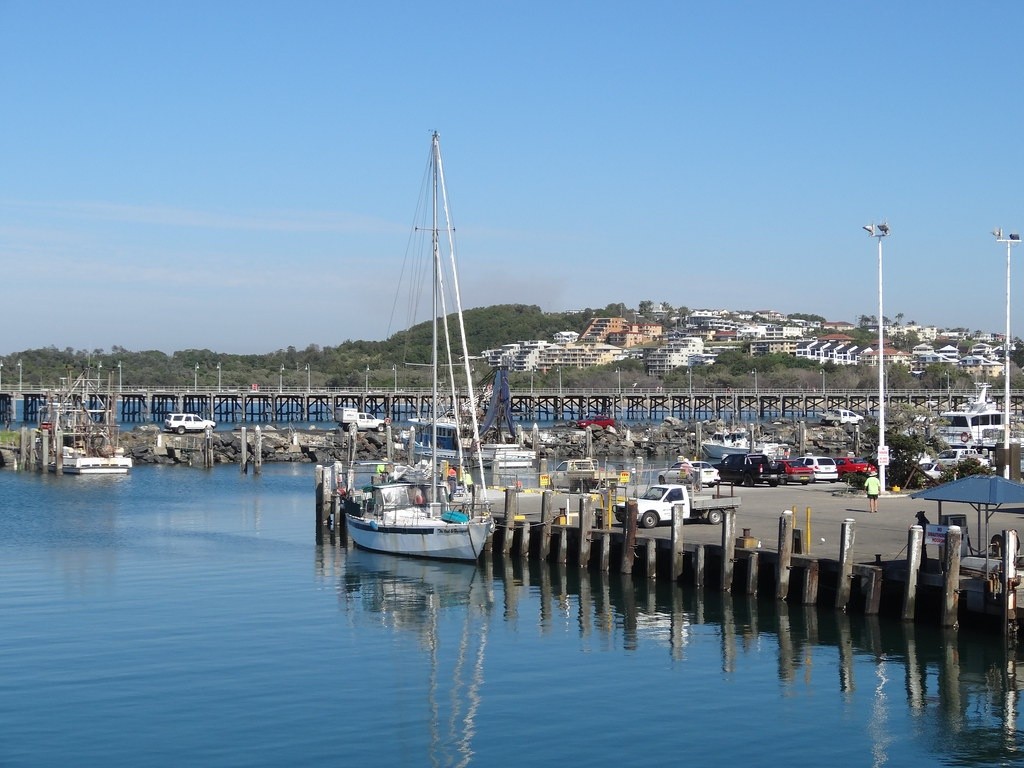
[551,458,619,494]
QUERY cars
[918,462,947,480]
[935,448,978,467]
[773,459,815,486]
[575,415,615,432]
[657,460,721,488]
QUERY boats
[37,443,133,475]
[402,416,537,471]
[926,380,1015,457]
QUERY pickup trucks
[817,409,865,428]
[611,483,742,529]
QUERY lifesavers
[384,418,392,425]
[961,432,969,443]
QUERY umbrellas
[909,474,1024,579]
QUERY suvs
[164,412,217,435]
[830,456,879,483]
[335,407,386,433]
[789,455,839,483]
[708,453,780,487]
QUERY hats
[684,458,689,463]
[382,458,388,461]
[870,471,878,476]
[915,511,925,517]
[452,463,457,467]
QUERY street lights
[118,360,122,392]
[392,363,397,394]
[279,363,285,393]
[97,360,103,392]
[944,369,949,395]
[751,368,758,396]
[304,362,311,394]
[615,366,621,394]
[819,368,826,395]
[686,367,692,395]
[194,361,200,392]
[556,365,562,394]
[531,365,536,393]
[216,361,222,393]
[992,226,1023,482]
[17,359,22,392]
[365,364,370,393]
[863,221,892,494]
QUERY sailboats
[340,128,497,566]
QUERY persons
[864,471,881,512]
[915,511,930,563]
[460,471,473,493]
[449,467,458,493]
